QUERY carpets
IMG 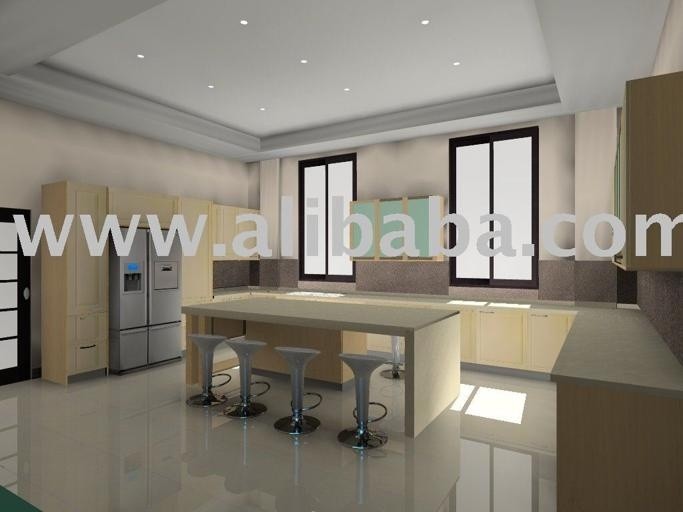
[0,487,41,511]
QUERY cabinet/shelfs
[213,203,261,261]
[610,71,683,273]
[108,188,178,229]
[349,194,443,260]
[527,310,575,382]
[551,309,683,512]
[178,197,213,351]
[41,179,109,385]
[428,305,476,371]
[475,308,528,377]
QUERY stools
[380,334,405,379]
[338,353,390,449]
[224,340,270,418]
[186,334,231,407]
[273,347,323,436]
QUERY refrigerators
[109,225,184,374]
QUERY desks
[181,297,461,438]
